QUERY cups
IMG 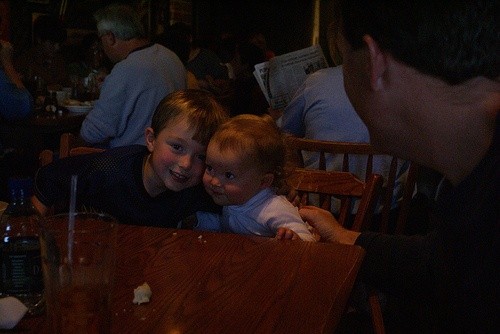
[38,212,118,334]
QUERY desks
[21,113,88,212]
[0,213,366,334]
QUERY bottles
[0,182,44,318]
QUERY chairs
[277,132,421,334]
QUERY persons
[297,0,500,334]
[0,0,416,244]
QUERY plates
[65,105,93,113]
